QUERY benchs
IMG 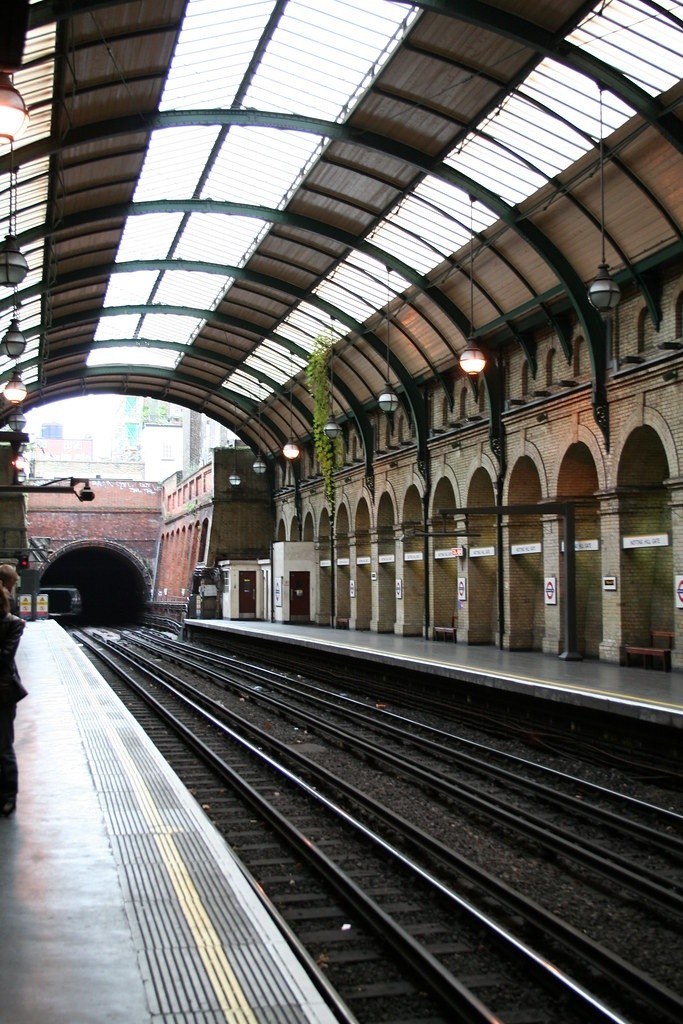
[434,615,458,644]
[624,631,679,672]
[336,617,349,627]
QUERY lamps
[252,380,266,476]
[322,315,343,441]
[0,68,33,476]
[586,89,622,321]
[458,193,487,377]
[282,351,301,459]
[228,404,242,486]
[377,265,401,413]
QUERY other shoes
[0,794,16,819]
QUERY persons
[0,562,28,815]
